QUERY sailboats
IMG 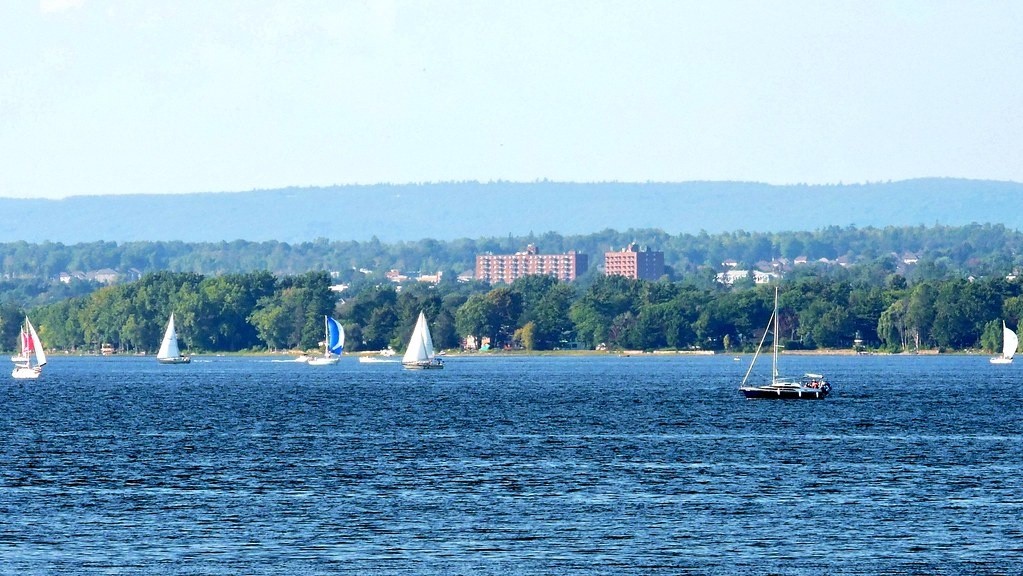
[738,286,832,400]
[401,308,447,370]
[989,319,1020,363]
[308,313,345,366]
[11,324,32,363]
[11,314,48,379]
[156,311,192,364]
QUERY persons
[802,379,820,390]
